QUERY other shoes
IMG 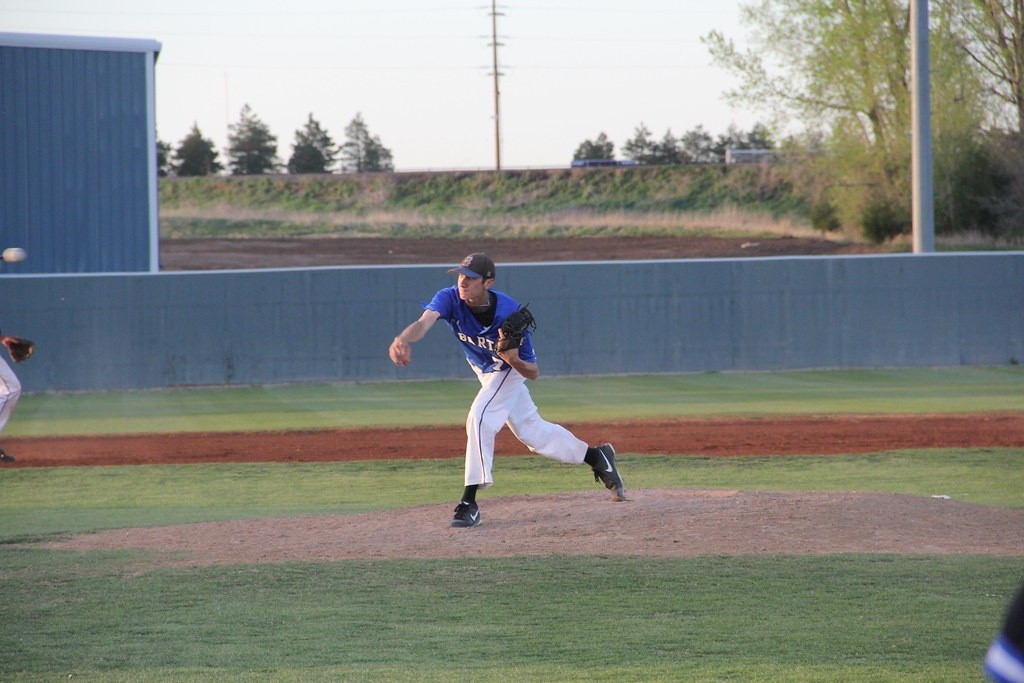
[0,449,15,461]
[982,635,1024,683]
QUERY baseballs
[3,247,27,263]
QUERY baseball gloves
[497,303,537,351]
[4,335,36,364]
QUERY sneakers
[451,497,481,528]
[592,443,624,501]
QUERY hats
[447,251,495,280]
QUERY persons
[389,252,624,529]
[984,578,1024,683]
[0,333,36,462]
